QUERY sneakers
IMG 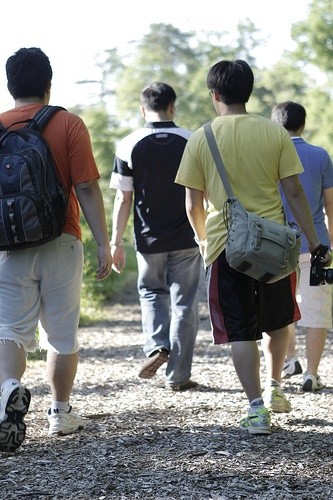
[239,406,271,434]
[0,382,31,452]
[269,386,292,413]
[280,358,302,379]
[47,405,83,436]
[302,374,323,390]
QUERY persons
[267,100,333,393]
[105,82,203,392]
[0,47,114,455]
[173,58,333,436]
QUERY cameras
[310,257,333,286]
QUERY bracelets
[108,238,123,246]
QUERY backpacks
[0,105,72,251]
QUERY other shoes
[168,381,197,391]
[138,349,168,378]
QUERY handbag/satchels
[223,197,301,284]
